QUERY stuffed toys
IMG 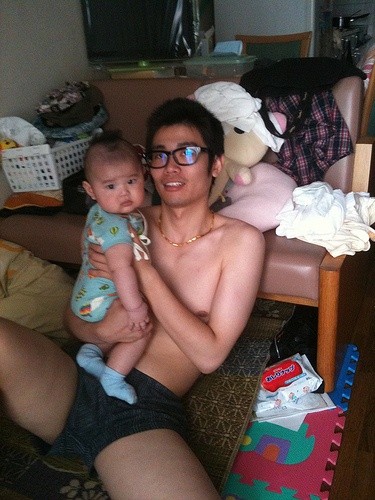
[1,138,18,149]
[190,83,287,206]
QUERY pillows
[215,162,300,233]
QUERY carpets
[0,302,294,500]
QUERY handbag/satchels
[241,53,368,138]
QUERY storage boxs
[1,136,91,192]
[108,53,258,80]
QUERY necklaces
[159,205,214,247]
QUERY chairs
[235,30,313,62]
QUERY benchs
[0,72,362,394]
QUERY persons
[70,134,152,404]
[0,97,264,499]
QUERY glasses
[143,145,212,170]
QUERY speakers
[81,0,215,67]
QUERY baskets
[1,136,92,192]
